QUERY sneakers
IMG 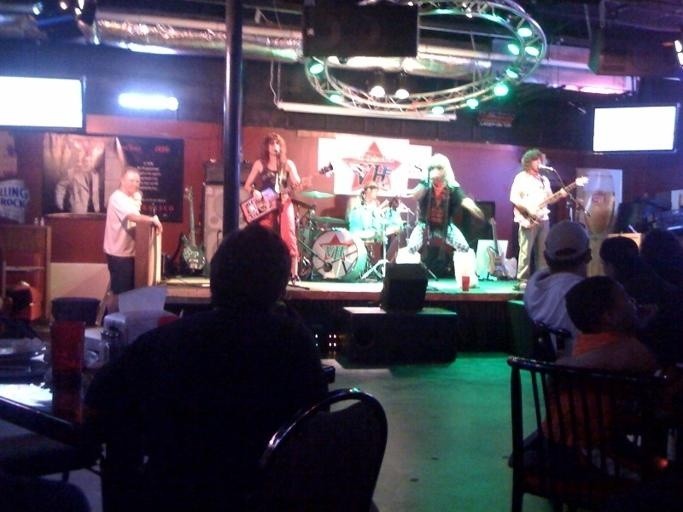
[289,274,302,287]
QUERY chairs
[0,252,42,338]
[267,389,388,512]
[507,357,661,512]
[530,320,571,363]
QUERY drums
[311,227,369,280]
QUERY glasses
[427,165,444,171]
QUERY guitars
[486,216,511,279]
[512,176,587,229]
[241,159,334,223]
[179,184,206,273]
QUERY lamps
[494,85,508,97]
[309,60,324,75]
[518,23,532,38]
[508,44,519,56]
[467,99,479,110]
[432,107,443,114]
[524,44,542,56]
[507,69,519,79]
[331,94,345,104]
[394,71,410,100]
[367,67,385,98]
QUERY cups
[51,375,81,422]
[51,319,84,377]
[462,276,470,291]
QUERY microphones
[537,164,554,173]
[273,143,281,155]
[414,165,425,172]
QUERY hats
[541,218,592,265]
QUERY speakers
[587,29,677,78]
[381,261,428,311]
[301,2,420,59]
[334,306,457,371]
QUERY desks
[1,339,109,476]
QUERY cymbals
[309,214,346,226]
[298,187,334,200]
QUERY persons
[104,167,165,311]
[87,226,332,511]
[54,155,100,214]
[522,228,683,474]
[509,149,568,289]
[406,153,496,275]
[345,184,382,228]
[244,131,300,281]
